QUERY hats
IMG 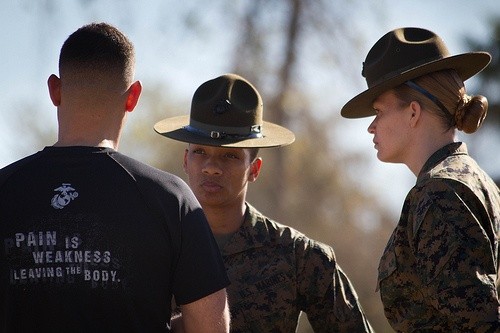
[153,74,295,148]
[341,27,492,119]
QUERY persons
[0,23,231,333]
[340,27,500,333]
[154,73,374,333]
